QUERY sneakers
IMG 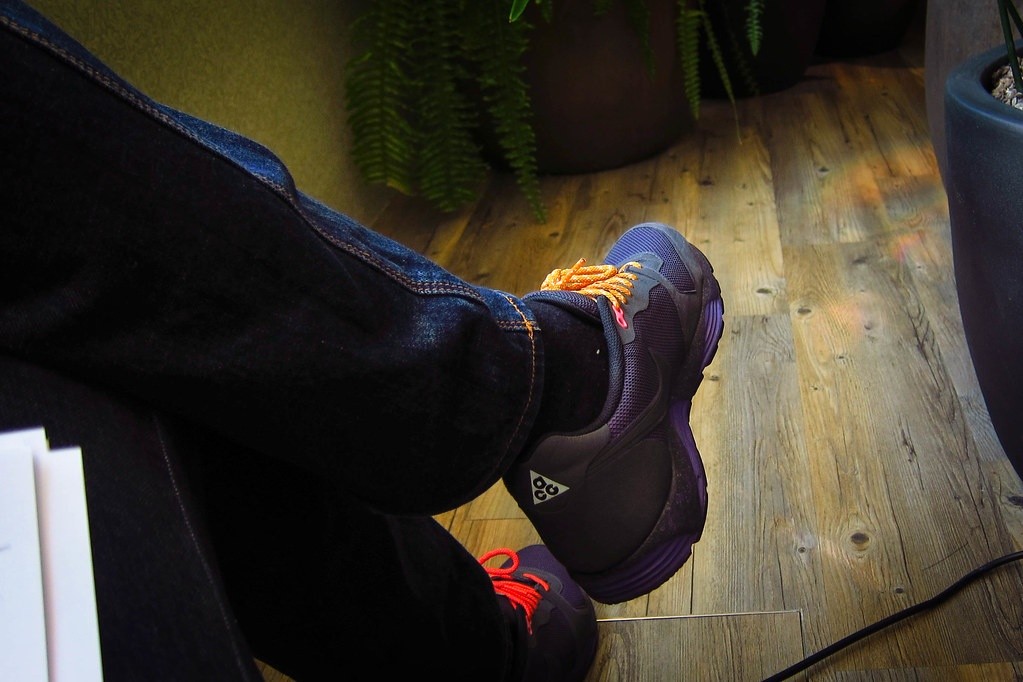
[489,542,598,682]
[501,223,727,604]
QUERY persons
[0,0,729,682]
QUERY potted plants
[944,0,1023,476]
[339,1,762,225]
